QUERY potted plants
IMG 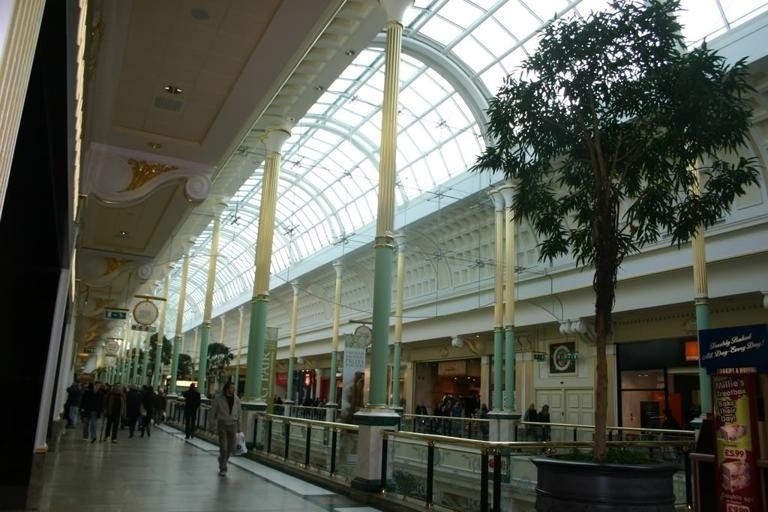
[468,0,766,512]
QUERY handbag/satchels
[232,430,249,456]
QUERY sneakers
[79,430,156,445]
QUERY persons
[211,381,243,477]
[342,371,365,418]
[658,408,678,464]
[274,392,552,454]
[62,380,168,444]
[182,383,201,440]
